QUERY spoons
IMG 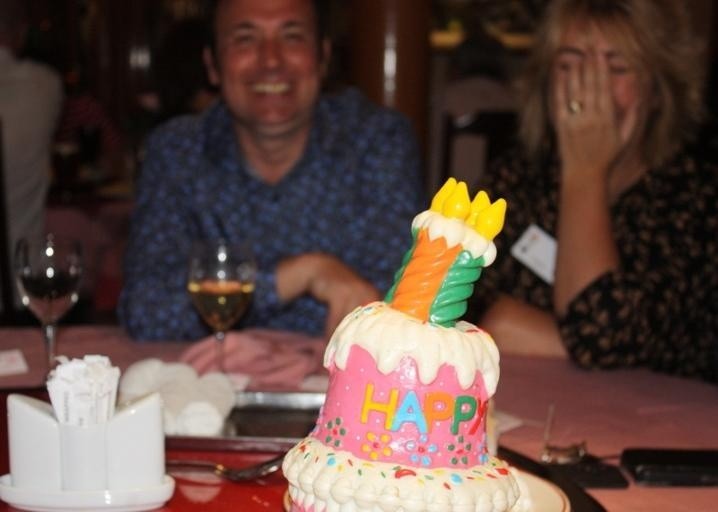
[168,449,284,482]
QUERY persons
[458,1,716,386]
[117,0,424,346]
[0,0,97,311]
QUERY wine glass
[13,229,88,385]
[185,239,256,371]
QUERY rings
[569,100,583,113]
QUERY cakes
[281,175,565,512]
